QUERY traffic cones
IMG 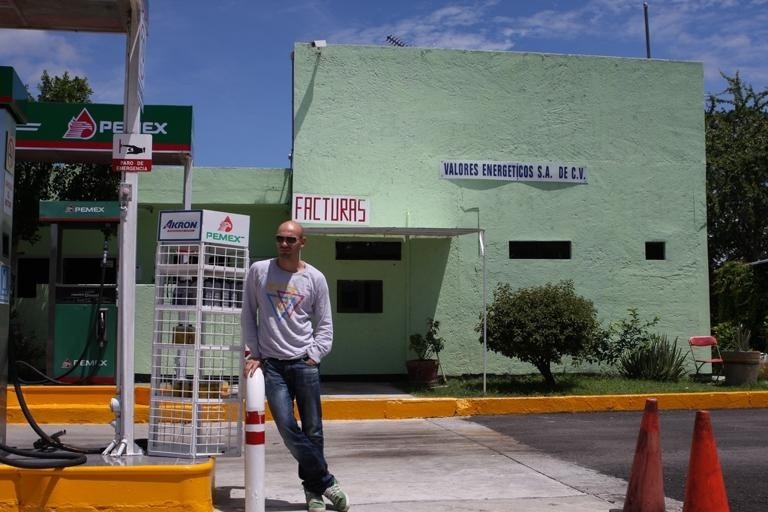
[681,410,731,512]
[609,397,674,511]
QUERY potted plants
[404,331,440,380]
[720,321,761,386]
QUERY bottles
[173,323,195,344]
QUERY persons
[239,221,350,512]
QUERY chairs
[688,336,727,385]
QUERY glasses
[276,235,297,243]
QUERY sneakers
[324,477,350,512]
[305,489,326,511]
